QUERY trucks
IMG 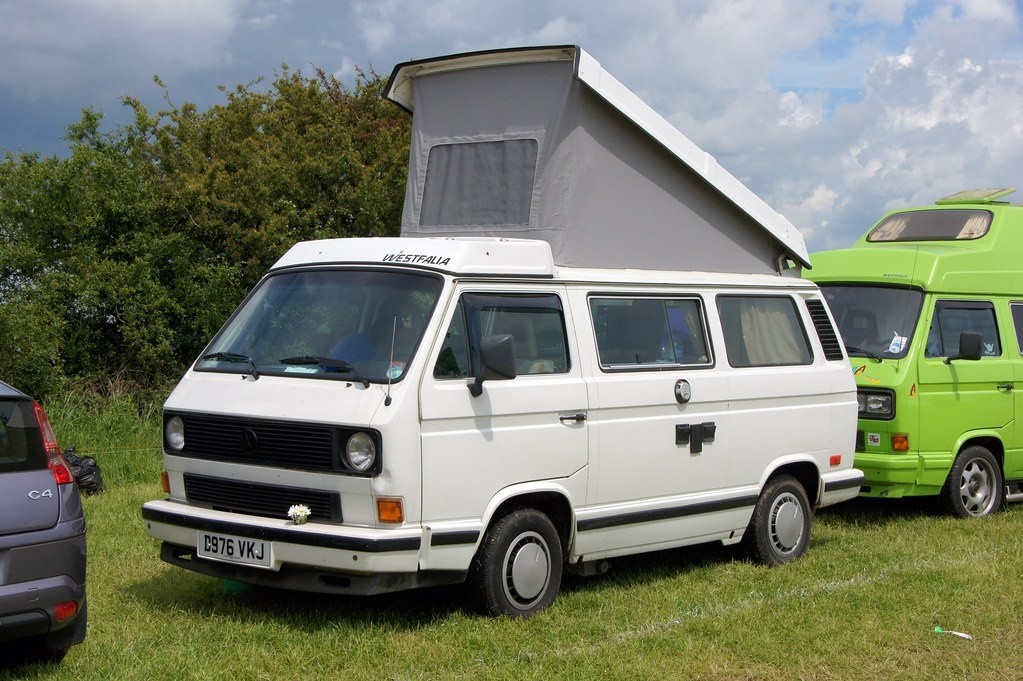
[776,186,1023,522]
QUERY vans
[141,44,869,623]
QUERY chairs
[491,314,554,374]
[369,299,425,364]
[836,307,882,348]
[934,311,977,350]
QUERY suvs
[0,379,89,665]
